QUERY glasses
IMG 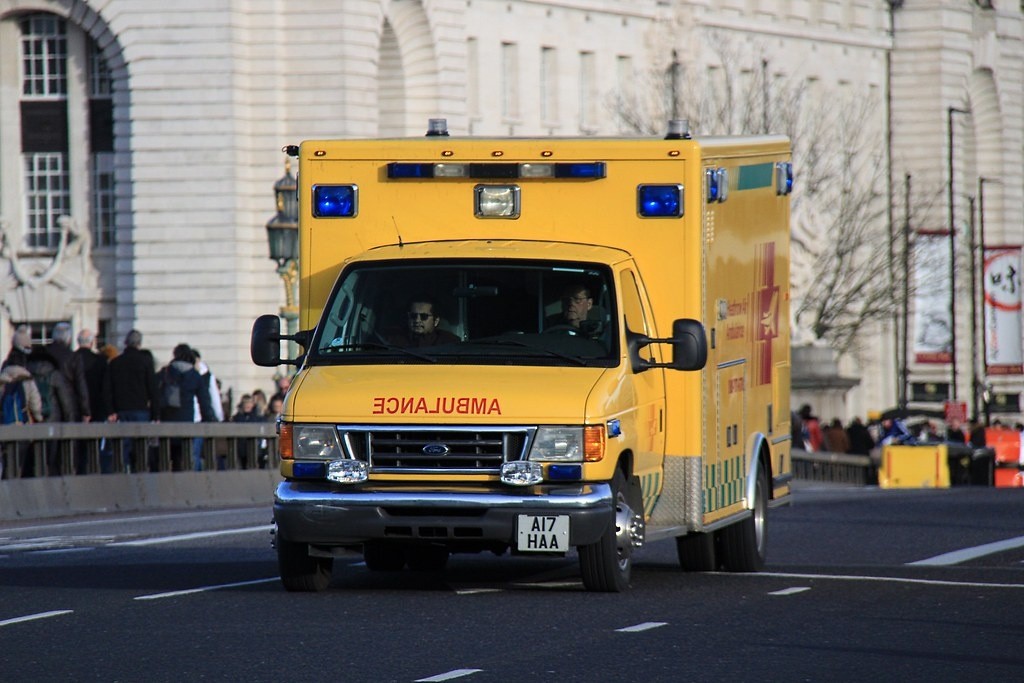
[410,312,433,320]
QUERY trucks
[251,134,794,594]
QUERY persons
[390,298,462,348]
[0,322,297,477]
[790,405,1024,487]
[539,280,599,337]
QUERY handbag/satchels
[161,383,180,409]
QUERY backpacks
[0,382,28,425]
[36,379,52,417]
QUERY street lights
[265,160,299,379]
[948,106,971,401]
[979,176,1000,372]
